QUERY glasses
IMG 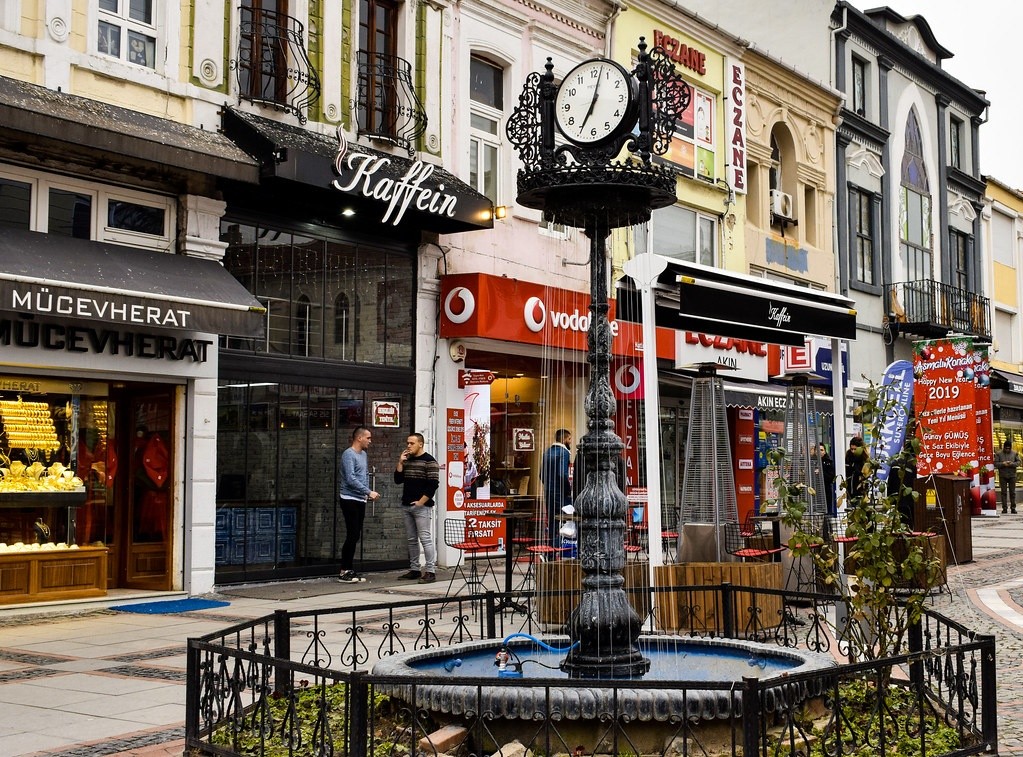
[1005,447,1010,450]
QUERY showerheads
[544,197,652,229]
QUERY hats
[1004,441,1011,447]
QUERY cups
[510,489,518,494]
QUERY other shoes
[1002,509,1007,513]
[1011,509,1017,513]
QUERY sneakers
[349,569,366,582]
[398,570,422,580]
[336,570,359,583]
[418,572,436,583]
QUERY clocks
[553,58,639,149]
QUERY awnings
[995,370,1023,394]
[221,106,494,235]
[0,225,267,339]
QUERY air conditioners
[769,189,792,222]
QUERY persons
[538,429,572,558]
[845,437,871,506]
[994,440,1021,513]
[789,441,833,514]
[339,427,380,582]
[393,433,440,584]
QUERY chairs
[444,508,951,627]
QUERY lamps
[342,208,356,217]
[494,206,507,219]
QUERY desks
[484,511,531,615]
[751,516,813,625]
